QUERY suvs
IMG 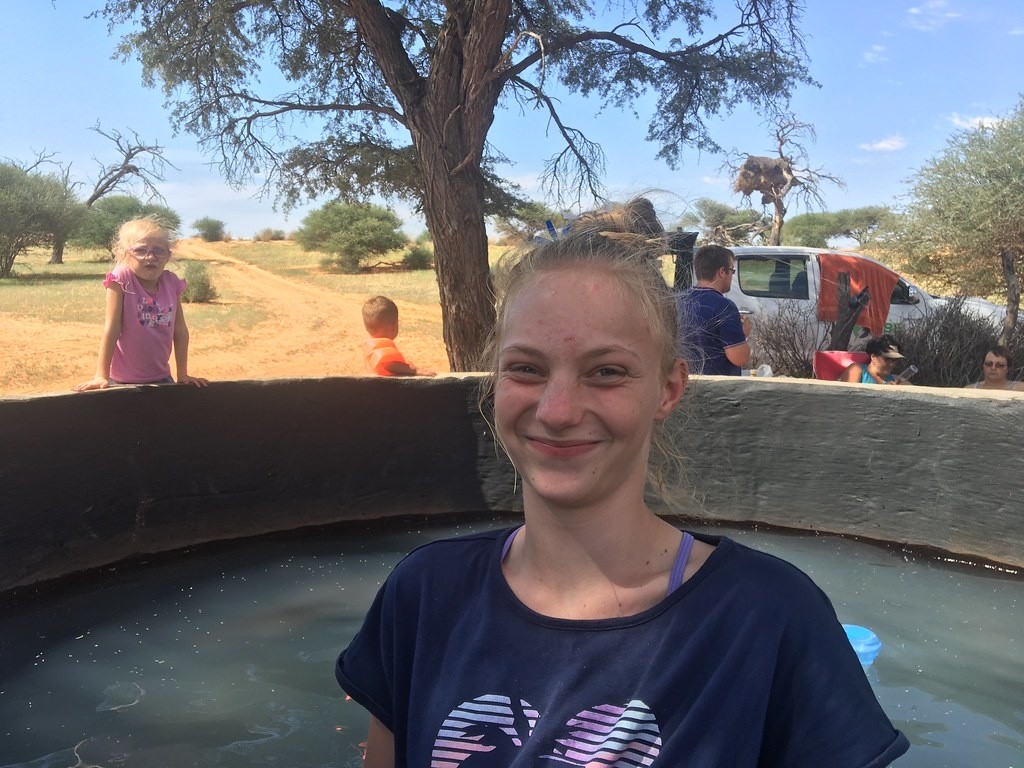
[690,246,1024,372]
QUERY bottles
[750,369,757,377]
[894,365,918,384]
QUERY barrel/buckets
[841,624,882,673]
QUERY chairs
[769,271,789,294]
[791,270,808,298]
[810,348,872,381]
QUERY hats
[879,345,907,359]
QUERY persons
[361,296,437,378]
[74,219,210,392]
[837,334,912,385]
[962,346,1024,392]
[674,245,753,377]
[333,232,910,768]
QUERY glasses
[129,247,170,260]
[721,267,736,275]
[984,361,1008,368]
